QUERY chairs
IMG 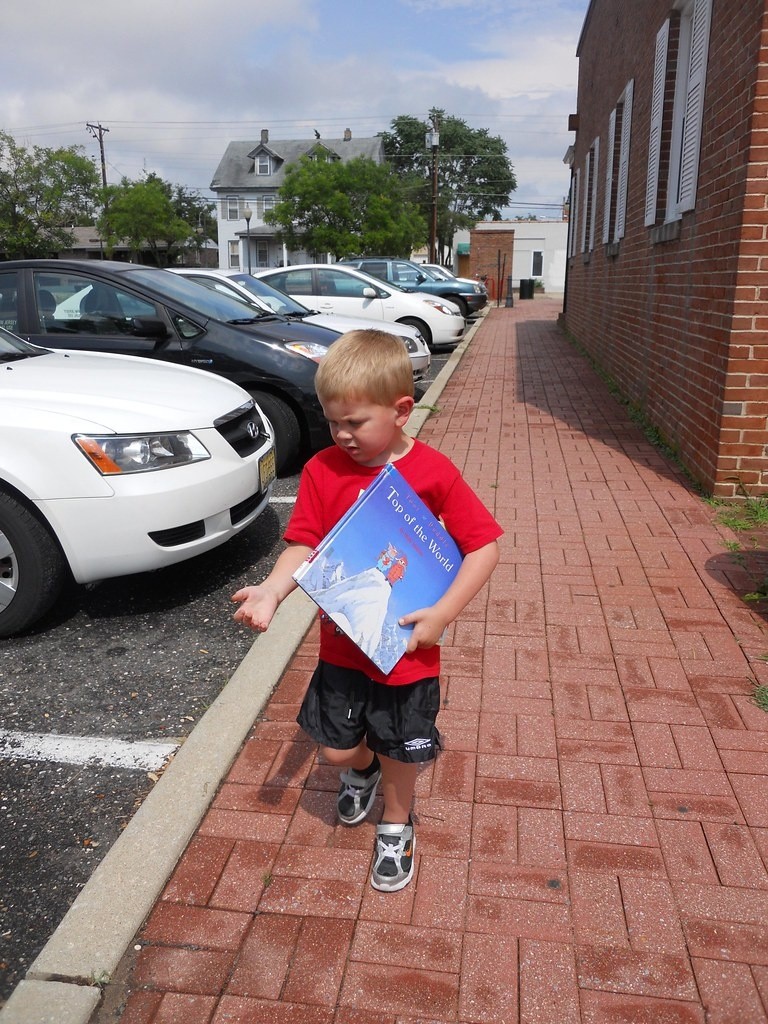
[38,290,58,332]
[317,273,331,295]
[78,288,125,335]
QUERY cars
[0,327,278,645]
[214,263,467,352]
[0,260,349,481]
[52,269,431,385]
[395,263,488,293]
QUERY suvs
[316,257,490,320]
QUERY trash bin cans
[519,279,534,299]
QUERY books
[293,464,463,674]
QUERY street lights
[196,210,207,267]
[242,202,253,276]
[89,239,107,260]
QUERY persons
[230,329,505,891]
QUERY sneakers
[371,814,417,893]
[335,762,380,825]
